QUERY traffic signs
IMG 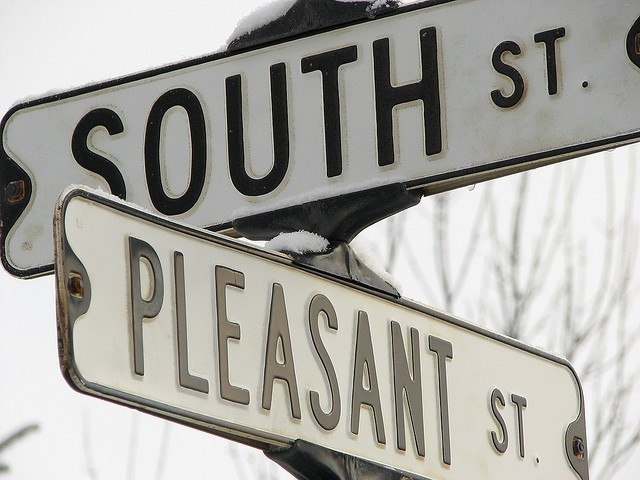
[0,0,639,280]
[52,180,592,480]
